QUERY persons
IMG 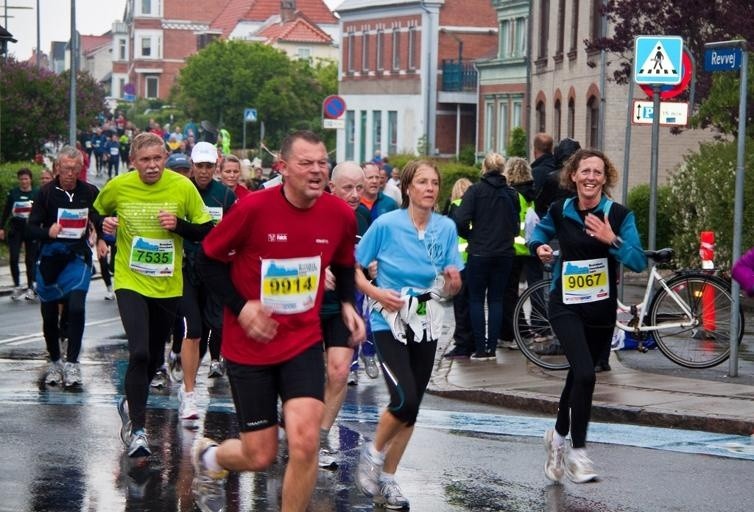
[81,111,230,301]
[189,128,368,512]
[450,131,580,360]
[730,244,754,297]
[353,158,462,511]
[1,145,98,389]
[147,142,283,420]
[315,159,445,467]
[528,147,647,485]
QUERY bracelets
[610,236,622,251]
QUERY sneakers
[544,427,567,486]
[104,292,116,301]
[176,383,200,420]
[117,395,133,448]
[347,348,379,386]
[443,329,565,362]
[151,350,184,388]
[564,446,600,485]
[127,426,152,458]
[44,355,84,387]
[354,439,385,498]
[319,447,340,467]
[371,476,410,510]
[11,286,36,302]
[207,359,223,379]
[189,437,229,512]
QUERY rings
[590,233,594,237]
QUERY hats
[189,141,218,164]
[165,152,192,170]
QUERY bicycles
[510,246,747,373]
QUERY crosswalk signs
[244,109,257,122]
[633,33,685,87]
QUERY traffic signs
[701,48,741,73]
